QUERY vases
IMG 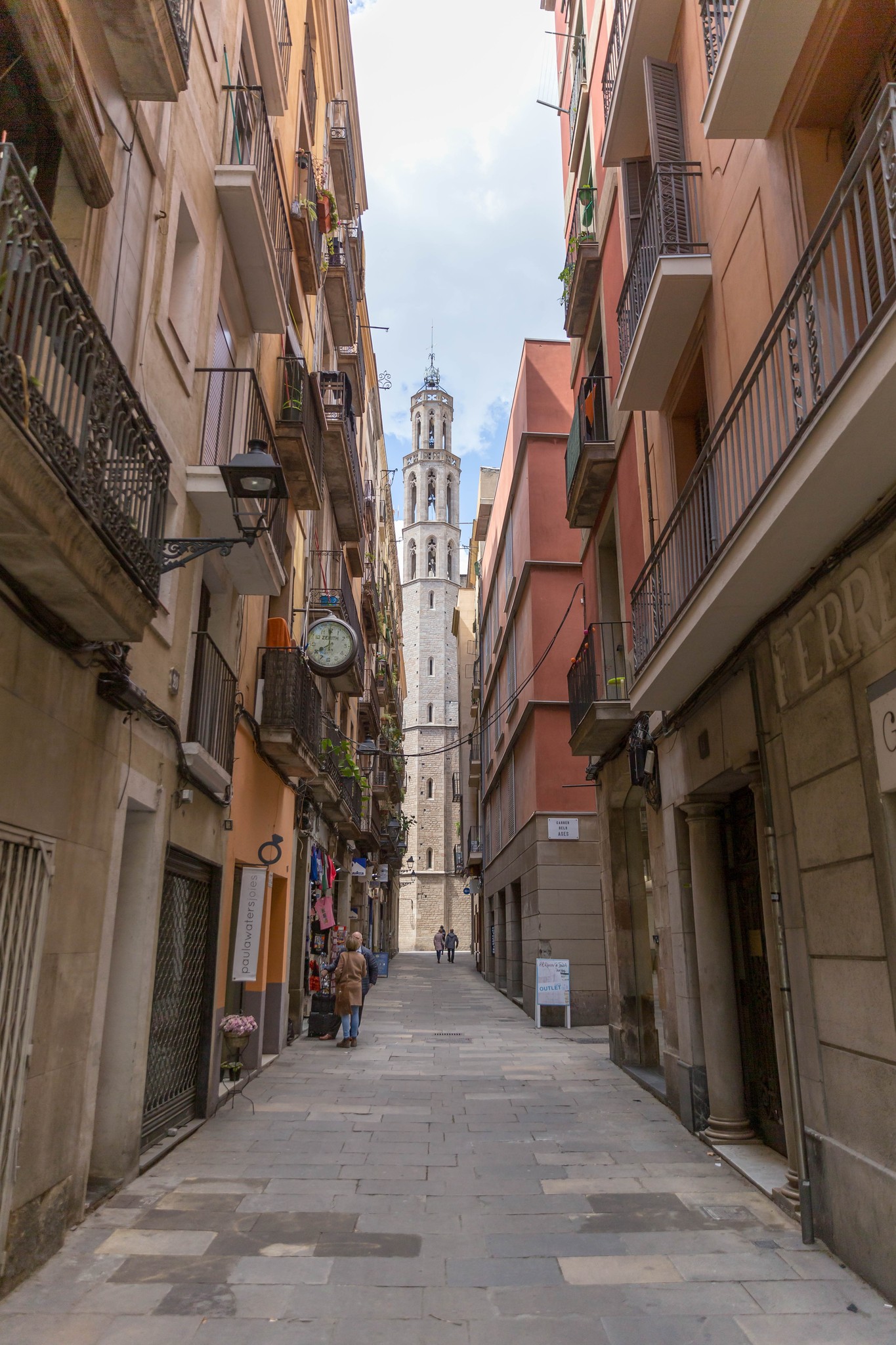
[362,581,371,591]
[298,156,308,169]
[317,195,332,233]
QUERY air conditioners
[378,771,386,785]
[471,841,477,852]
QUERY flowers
[219,1015,258,1035]
[321,188,340,233]
[297,147,329,189]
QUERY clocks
[300,612,358,677]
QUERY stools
[604,677,626,701]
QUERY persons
[445,929,459,963]
[334,937,367,1048]
[319,932,378,1041]
[434,929,444,963]
[440,925,446,955]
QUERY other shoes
[441,952,444,955]
[351,1037,357,1047]
[437,961,440,964]
[447,958,451,962]
[336,1036,350,1048]
[452,962,454,963]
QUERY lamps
[587,765,598,780]
[224,819,233,831]
[181,790,193,804]
[387,816,416,888]
[357,735,379,778]
[109,442,291,574]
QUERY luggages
[308,972,337,1038]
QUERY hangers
[321,893,325,898]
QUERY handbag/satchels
[333,984,353,1015]
[311,988,336,1014]
[440,934,444,945]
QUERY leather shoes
[319,1033,336,1040]
[349,1036,352,1040]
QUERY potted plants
[220,1061,243,1082]
[380,713,392,724]
[375,672,385,680]
[376,655,388,665]
[558,184,594,305]
